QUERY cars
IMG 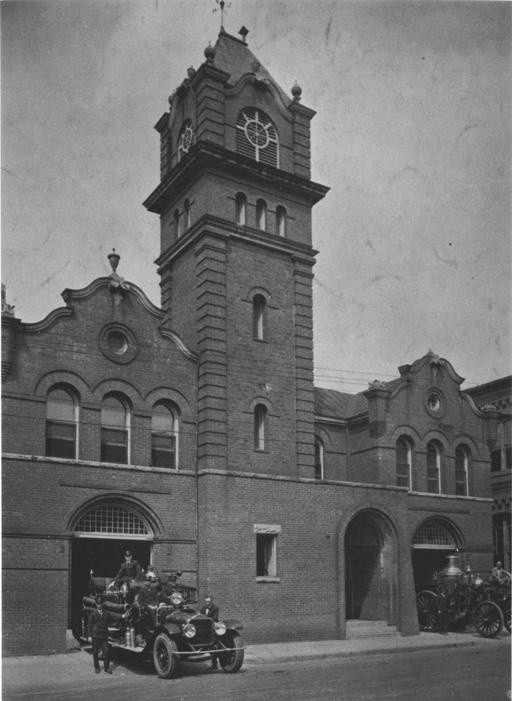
[416,554,511,638]
[79,568,248,679]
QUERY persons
[107,550,143,591]
[87,597,127,674]
[488,560,511,584]
[201,597,220,670]
[435,588,450,635]
